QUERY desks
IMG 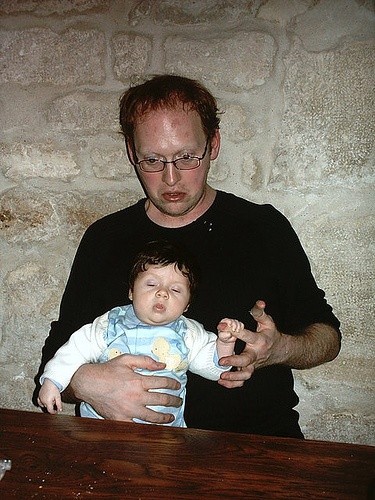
[0,408,375,500]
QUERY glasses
[131,136,209,173]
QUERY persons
[37,240,244,427]
[31,75,344,441]
